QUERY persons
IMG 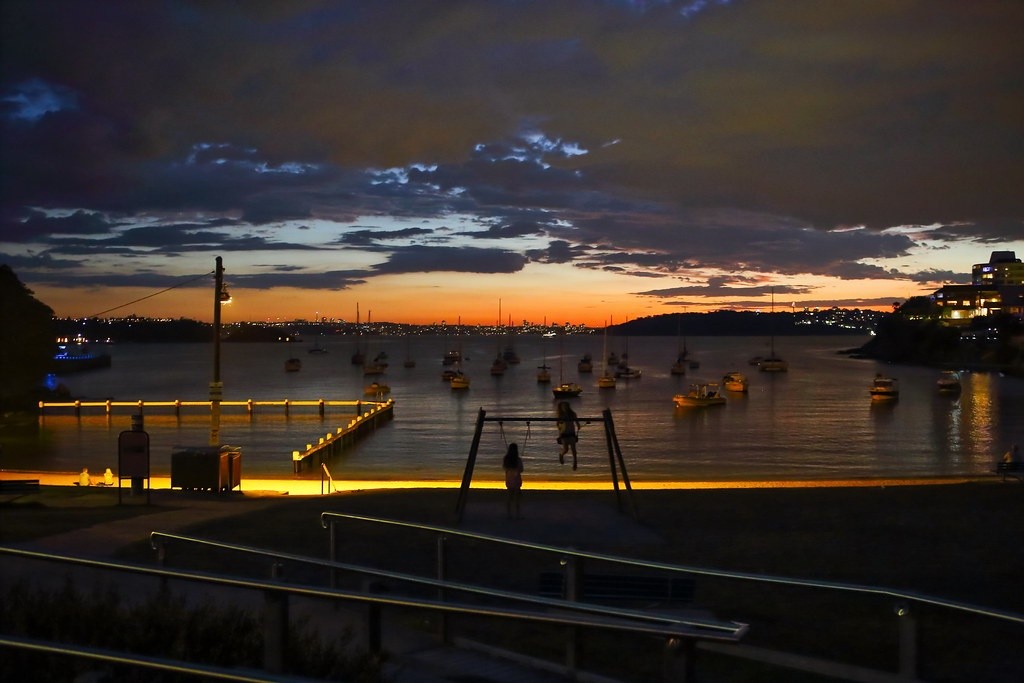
[555,402,580,471]
[1002,443,1021,464]
[502,442,525,521]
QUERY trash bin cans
[170,442,243,497]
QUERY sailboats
[402,329,415,370]
[552,328,581,399]
[536,315,553,384]
[307,325,327,355]
[756,284,790,370]
[668,305,703,377]
[350,302,366,363]
[437,298,470,390]
[490,297,520,378]
[362,308,389,376]
[595,314,641,389]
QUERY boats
[44,351,115,374]
[578,351,593,374]
[671,383,726,411]
[287,358,301,372]
[935,369,961,396]
[869,375,900,403]
[722,370,746,393]
